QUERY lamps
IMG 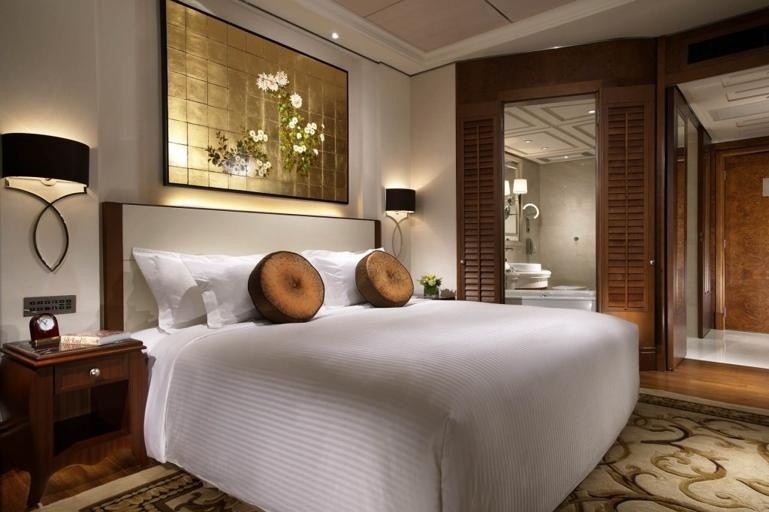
[0,133,89,273]
[504,179,527,219]
[385,189,415,258]
[504,180,510,197]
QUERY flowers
[416,273,443,295]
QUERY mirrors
[504,152,524,249]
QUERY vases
[424,285,439,299]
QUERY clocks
[30,312,60,347]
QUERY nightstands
[0,332,149,506]
[412,295,456,300]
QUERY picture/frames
[160,0,349,204]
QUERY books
[60,328,131,347]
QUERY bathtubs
[504,289,596,311]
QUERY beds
[102,200,641,512]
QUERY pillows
[131,247,414,335]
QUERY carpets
[28,387,769,512]
